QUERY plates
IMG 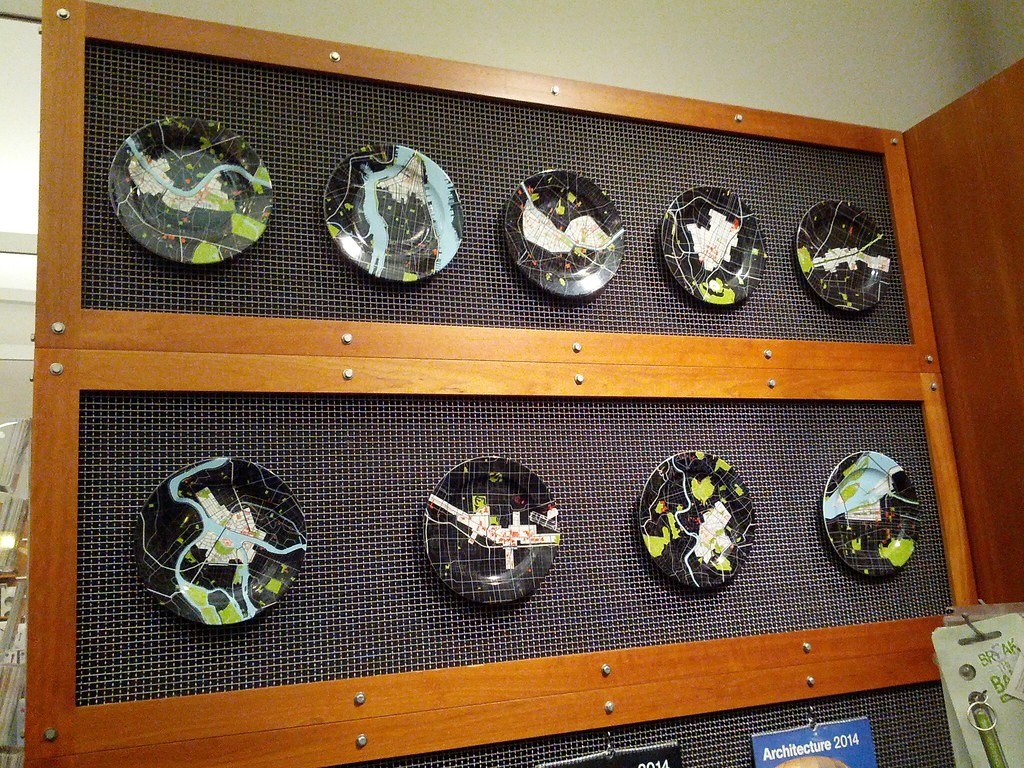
[796,198,892,311]
[107,116,273,264]
[662,187,766,305]
[134,457,307,625]
[424,456,561,604]
[823,451,921,576]
[323,143,464,282]
[639,451,756,588]
[504,169,624,297]
